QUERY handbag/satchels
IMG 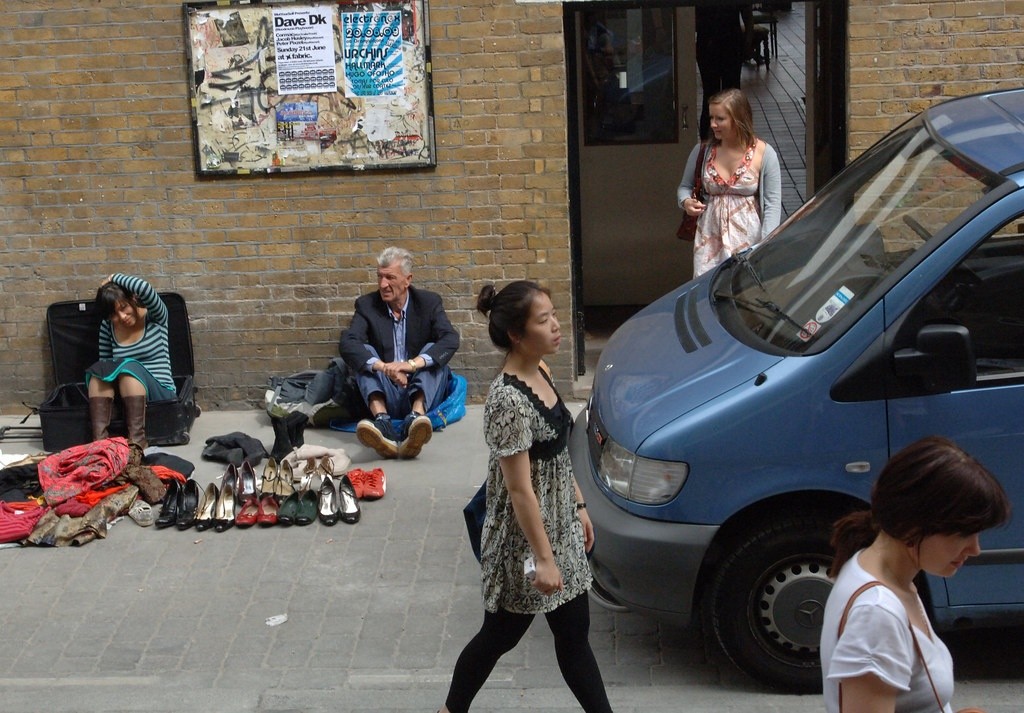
[463,478,486,565]
[264,358,360,429]
[280,443,351,482]
[677,143,708,243]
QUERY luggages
[0,292,201,452]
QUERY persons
[339,247,459,459]
[678,89,782,278]
[437,281,614,713]
[694,0,756,144]
[86,272,176,458]
[819,435,1012,713]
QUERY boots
[268,411,309,464]
[201,431,269,468]
[87,396,114,444]
[122,394,149,453]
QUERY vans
[569,82,1024,695]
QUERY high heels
[259,456,297,505]
[195,483,237,532]
[319,475,361,525]
[298,454,335,500]
[154,478,198,530]
[220,461,257,504]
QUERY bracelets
[408,359,417,372]
[108,273,114,282]
[577,502,586,510]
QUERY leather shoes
[279,489,318,525]
[235,496,279,527]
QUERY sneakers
[398,415,433,458]
[356,419,399,459]
[127,499,154,527]
[344,468,386,499]
